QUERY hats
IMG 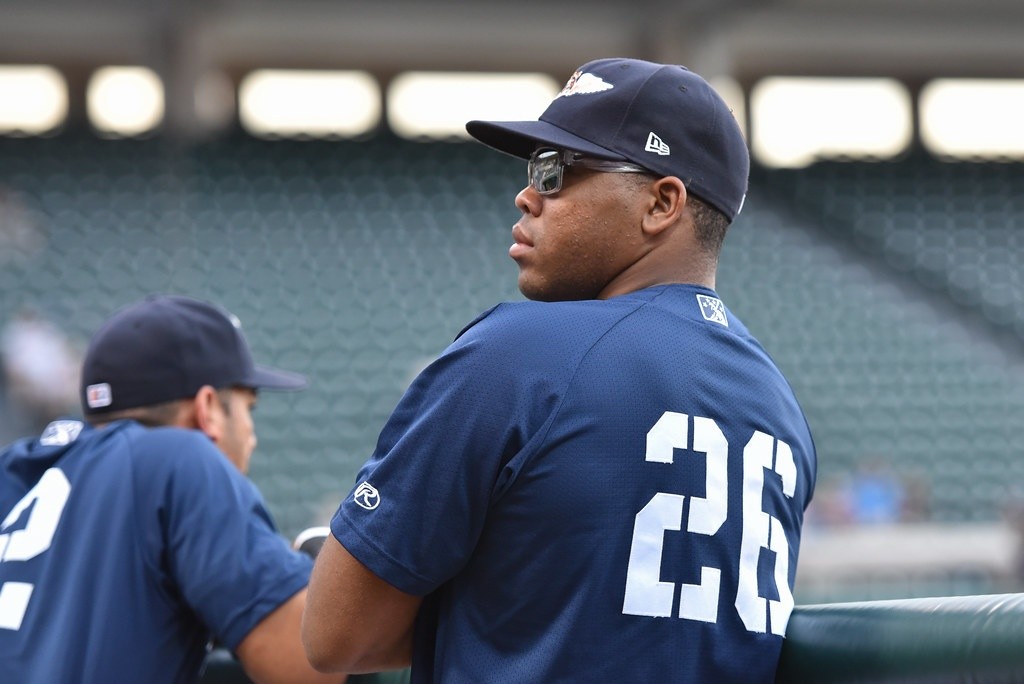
[465,58,751,223]
[80,296,307,415]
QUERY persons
[301,56,813,684]
[0,299,341,684]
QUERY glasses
[527,147,646,195]
